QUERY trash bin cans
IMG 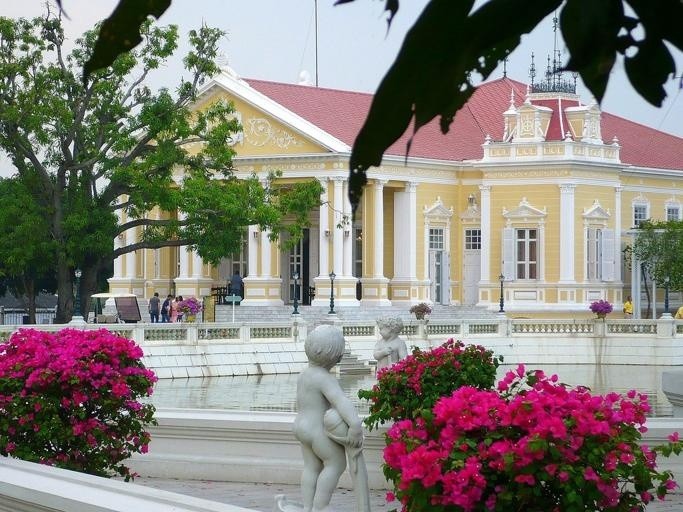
[23,315,29,324]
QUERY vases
[596,312,606,318]
[185,314,195,321]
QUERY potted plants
[408,302,431,320]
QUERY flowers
[588,300,613,314]
[174,296,206,316]
[0,323,158,484]
[356,336,681,510]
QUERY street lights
[292,272,300,314]
[74,267,82,316]
[664,276,670,313]
[499,274,505,312]
[329,270,336,314]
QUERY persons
[292,325,364,512]
[148,292,160,323]
[162,295,185,323]
[623,296,633,331]
[374,313,409,369]
[229,271,242,305]
[674,307,683,331]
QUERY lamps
[290,269,336,315]
